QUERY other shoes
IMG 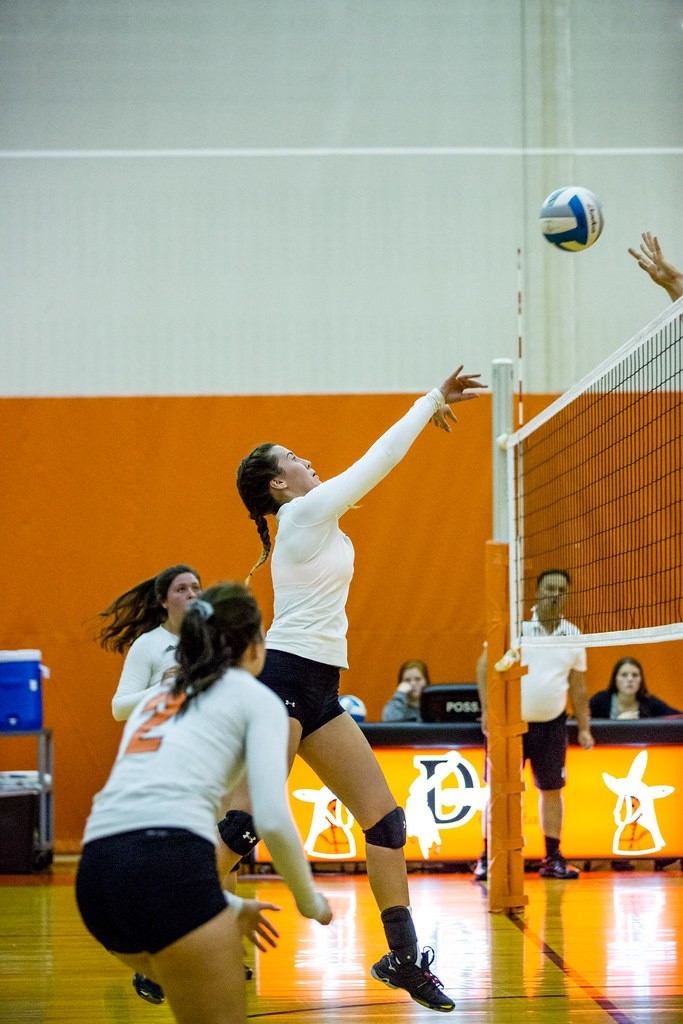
[610,860,636,870]
[243,963,254,980]
[653,858,680,871]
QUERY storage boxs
[0,650,52,732]
[0,770,52,874]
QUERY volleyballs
[539,183,604,253]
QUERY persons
[628,230,683,303]
[381,658,432,723]
[79,565,254,981]
[474,568,595,880]
[589,657,683,721]
[74,583,333,1024]
[131,364,489,1013]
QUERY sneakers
[539,849,579,879]
[473,852,488,881]
[371,946,456,1013]
[132,971,166,1005]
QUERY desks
[241,720,683,874]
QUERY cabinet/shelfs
[0,729,53,867]
